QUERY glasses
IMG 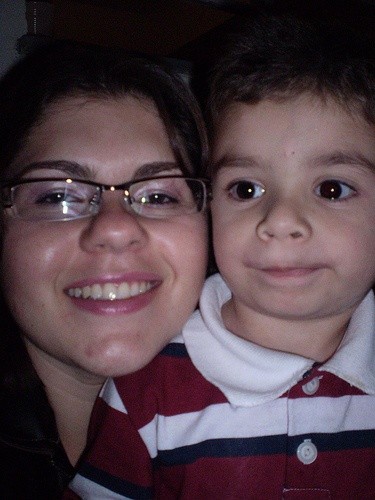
[0,175,213,223]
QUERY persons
[0,43,215,500]
[62,14,375,500]
